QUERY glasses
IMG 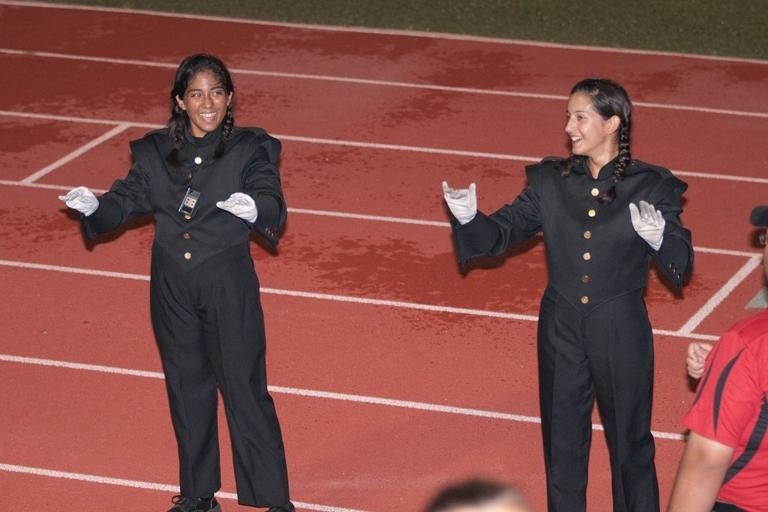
[753,229,766,247]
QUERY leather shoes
[171,497,294,510]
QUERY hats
[751,207,768,225]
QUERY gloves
[58,186,99,216]
[216,192,258,223]
[629,200,665,251]
[442,181,477,226]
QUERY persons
[424,478,531,512]
[685,206,767,380]
[57,54,296,512]
[443,77,694,512]
[667,311,768,512]
